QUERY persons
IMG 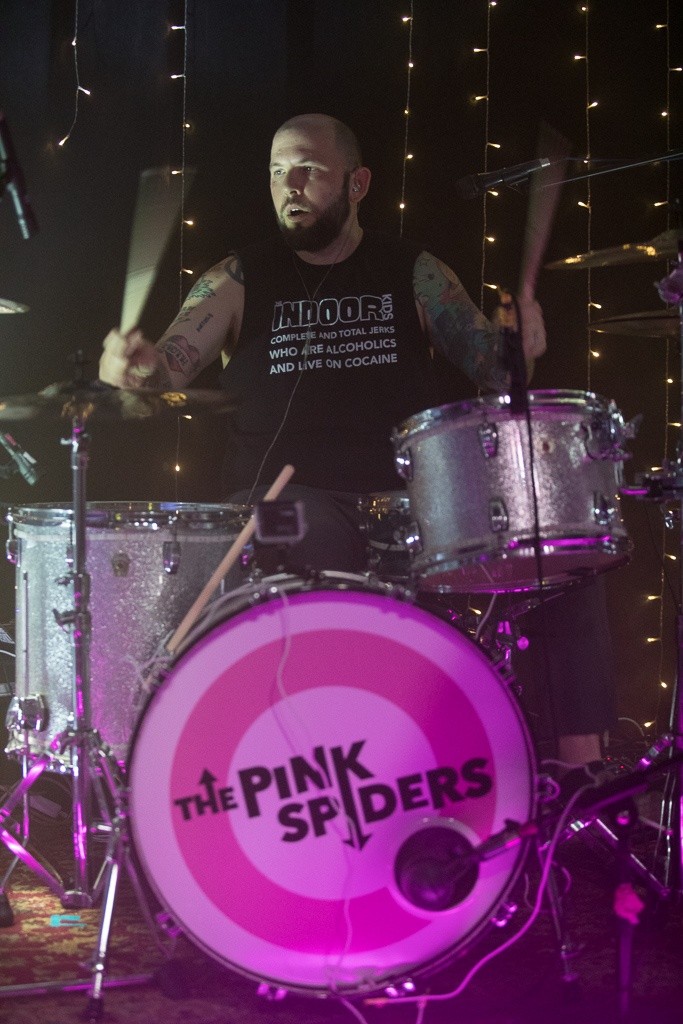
[98,114,642,982]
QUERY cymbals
[543,229,683,272]
[0,380,230,424]
[589,309,683,337]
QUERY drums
[125,568,539,997]
[390,388,639,595]
[4,500,265,778]
